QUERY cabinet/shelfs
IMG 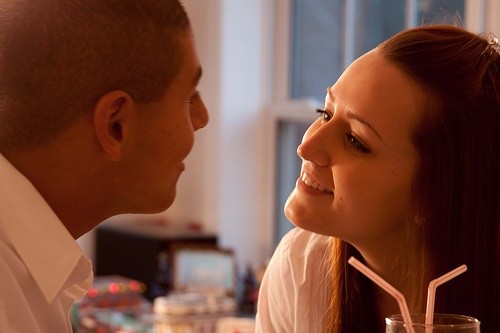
[95,225,218,302]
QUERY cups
[385,313,481,333]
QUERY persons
[255,25,499,333]
[0,0,209,333]
